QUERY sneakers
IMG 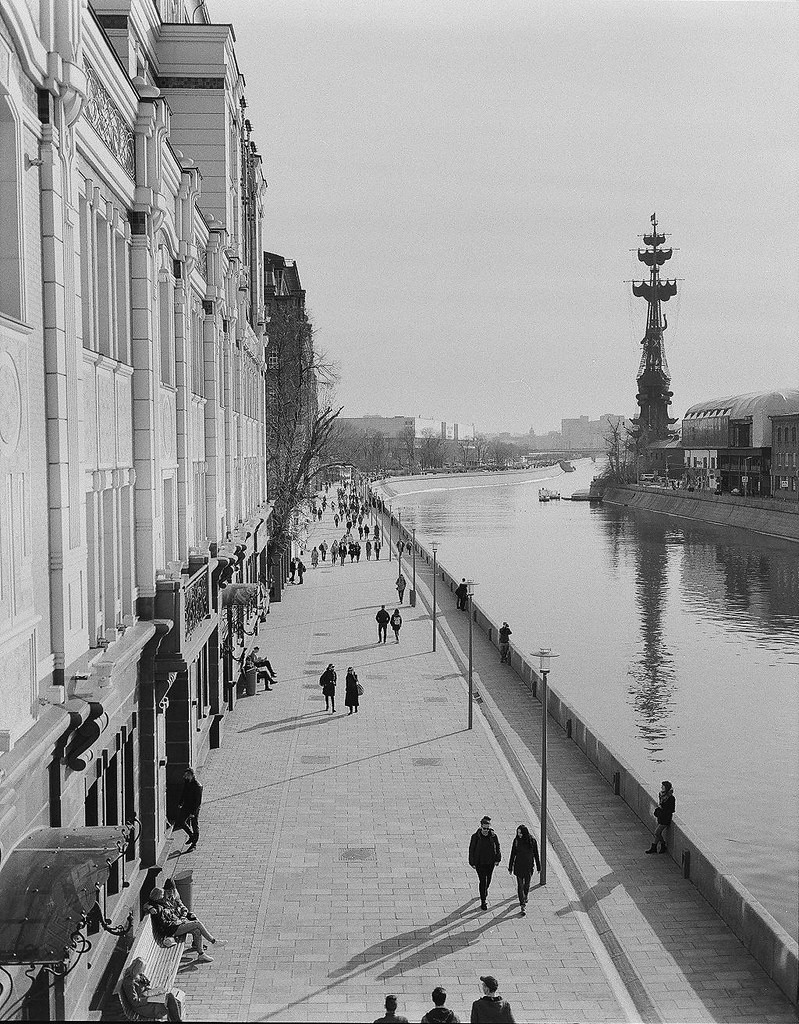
[198,953,214,962]
[213,939,228,948]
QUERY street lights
[745,457,752,498]
[462,579,479,729]
[410,522,419,607]
[666,455,672,490]
[636,455,643,485]
[530,648,560,885]
[428,541,441,652]
[395,507,404,576]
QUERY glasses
[482,827,489,830]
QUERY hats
[164,878,176,890]
[163,937,177,947]
[480,976,498,990]
[150,888,162,901]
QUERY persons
[143,877,228,961]
[289,557,304,585]
[508,824,541,915]
[311,477,394,567]
[320,664,359,714]
[421,987,460,1024]
[469,816,501,910]
[373,995,408,1024]
[396,574,406,604]
[376,605,402,644]
[499,621,512,661]
[243,646,278,691]
[471,975,515,1024]
[396,539,413,555]
[177,768,204,851]
[645,780,676,853]
[455,578,468,611]
[123,957,182,1022]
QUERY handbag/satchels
[654,808,660,817]
[303,566,306,572]
[357,684,364,695]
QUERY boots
[521,903,528,915]
[645,843,658,853]
[660,842,667,853]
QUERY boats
[562,490,603,501]
[663,526,684,543]
[538,487,561,502]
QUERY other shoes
[332,710,336,713]
[185,834,194,844]
[326,706,328,710]
[272,673,277,677]
[348,712,352,715]
[192,942,207,950]
[270,680,277,684]
[265,688,272,691]
[187,844,196,853]
[355,710,358,713]
[482,901,487,910]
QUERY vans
[641,474,654,482]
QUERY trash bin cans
[409,589,416,605]
[173,870,193,911]
[246,673,258,696]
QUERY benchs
[111,912,184,1020]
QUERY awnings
[222,583,261,662]
[0,819,143,1021]
